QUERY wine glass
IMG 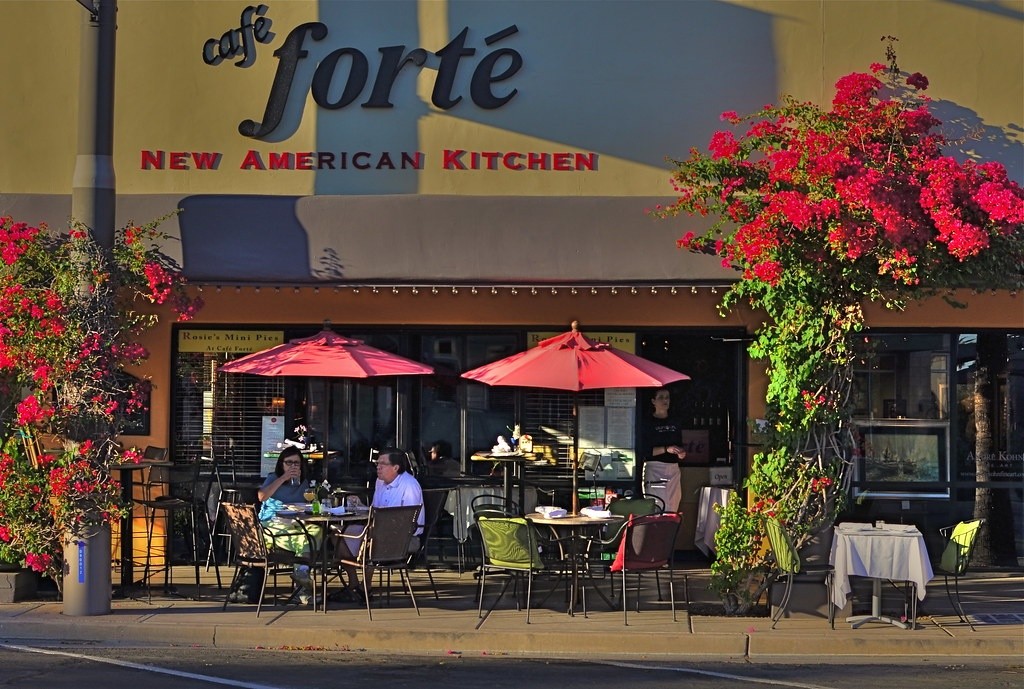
[303,489,315,504]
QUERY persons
[258,446,329,604]
[639,388,686,568]
[332,453,425,601]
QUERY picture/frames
[850,416,950,500]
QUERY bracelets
[665,446,667,453]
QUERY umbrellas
[461,321,690,606]
[215,330,433,595]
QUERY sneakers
[291,590,316,605]
[290,569,313,590]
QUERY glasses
[374,460,391,467]
[428,451,434,453]
[283,460,302,467]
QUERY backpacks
[227,565,266,604]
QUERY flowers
[0,216,207,580]
[643,26,1024,523]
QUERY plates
[276,510,298,515]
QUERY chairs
[760,512,838,630]
[117,445,685,625]
[900,517,987,633]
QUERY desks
[106,458,174,600]
[263,449,340,481]
[471,451,556,518]
[446,474,538,571]
[332,478,375,506]
[829,522,933,632]
[524,510,627,619]
[695,484,738,556]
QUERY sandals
[327,585,374,603]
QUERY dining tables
[276,502,372,613]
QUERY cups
[291,470,300,486]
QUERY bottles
[312,499,320,515]
[321,499,332,516]
